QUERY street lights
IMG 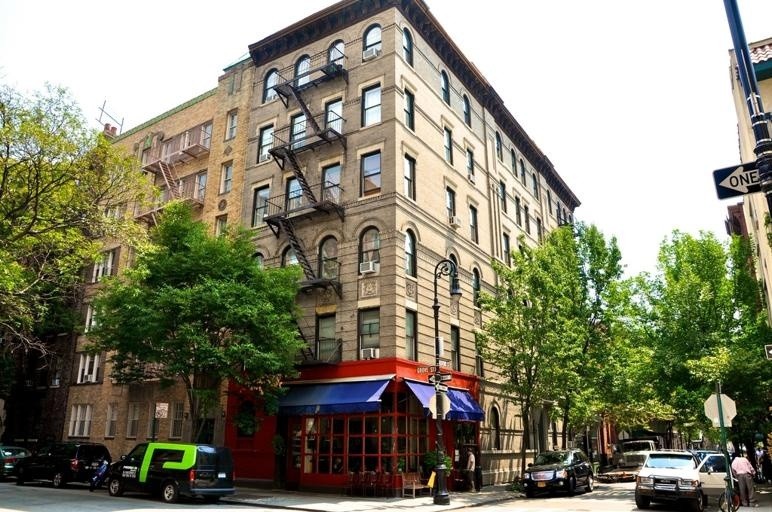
[433,258,463,506]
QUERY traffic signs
[428,373,451,392]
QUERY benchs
[401,472,430,498]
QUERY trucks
[616,436,666,469]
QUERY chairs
[341,470,395,497]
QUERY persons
[730,451,759,508]
[757,453,772,487]
[465,447,478,492]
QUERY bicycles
[718,476,740,512]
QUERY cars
[522,449,594,498]
[636,449,734,512]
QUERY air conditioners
[360,262,376,274]
[450,216,462,228]
[468,173,475,184]
[362,47,378,61]
[362,349,376,359]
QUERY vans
[0,441,236,503]
